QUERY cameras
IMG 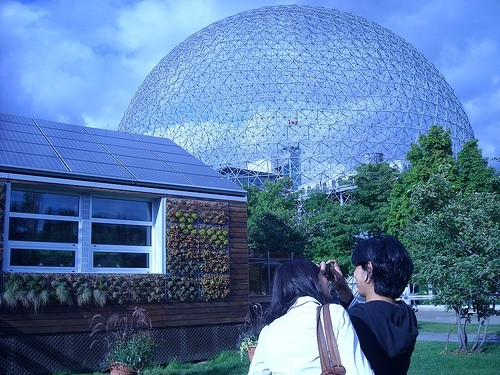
[323,263,334,280]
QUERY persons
[247,260,375,375]
[316,235,419,375]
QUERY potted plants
[240,337,258,362]
[106,335,157,375]
